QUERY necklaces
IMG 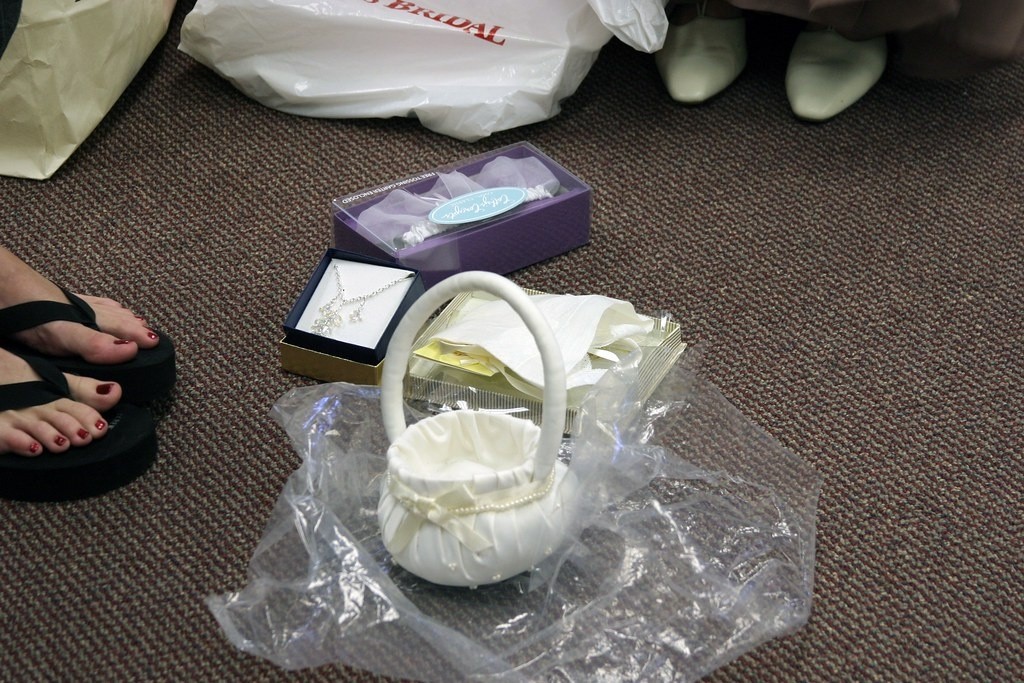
[310,265,408,336]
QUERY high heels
[0,346,157,501]
[0,279,175,404]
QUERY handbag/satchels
[376,270,585,589]
[177,0,668,145]
[0,0,177,181]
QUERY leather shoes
[785,26,887,122]
[655,0,748,105]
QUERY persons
[0,245,175,499]
[654,0,1024,123]
